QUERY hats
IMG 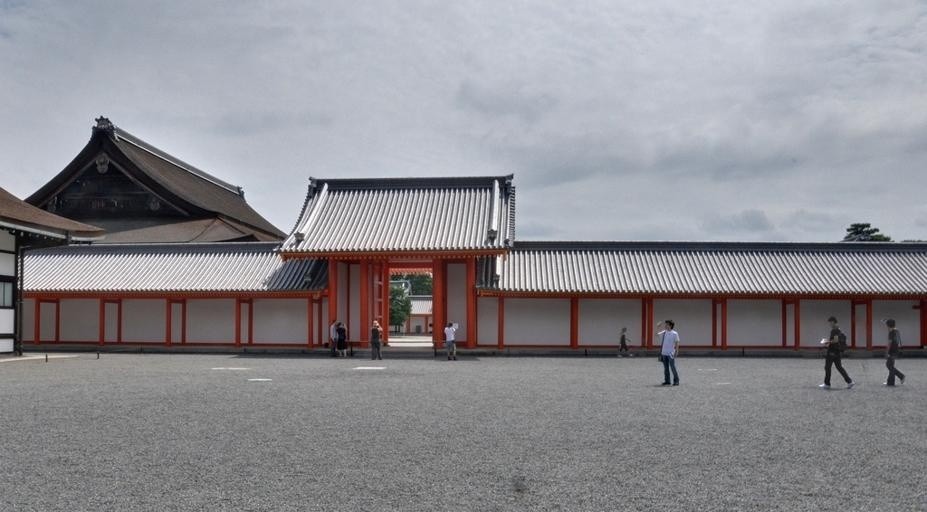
[371,321,379,326]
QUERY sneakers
[883,382,888,386]
[845,382,855,388]
[901,376,906,384]
[662,382,678,386]
[819,384,831,388]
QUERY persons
[329,318,339,357]
[818,316,856,390]
[335,321,349,358]
[879,319,906,387]
[617,327,633,358]
[443,321,458,361]
[369,319,384,360]
[655,320,680,386]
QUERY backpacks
[834,328,847,352]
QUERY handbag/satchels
[658,352,663,362]
[897,349,904,359]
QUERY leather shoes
[448,356,456,360]
